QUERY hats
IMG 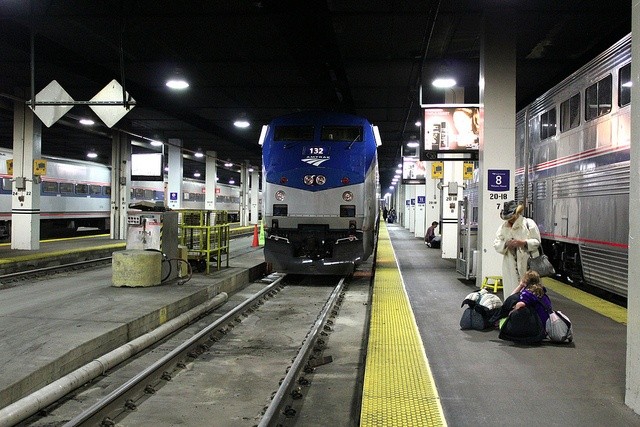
[501,200,523,221]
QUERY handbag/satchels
[499,305,548,344]
[546,310,573,345]
[460,308,490,331]
[527,239,555,278]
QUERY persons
[383,207,387,222]
[505,269,553,339]
[493,200,541,301]
[424,221,438,243]
